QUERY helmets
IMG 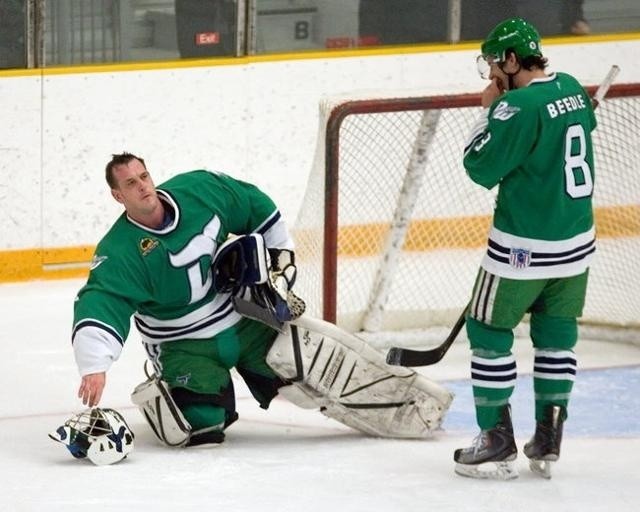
[48,405,135,464]
[479,17,543,62]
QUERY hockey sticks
[385,65,620,367]
[230,290,286,336]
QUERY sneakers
[521,421,563,461]
[451,420,518,466]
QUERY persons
[69,150,458,455]
[452,17,601,468]
